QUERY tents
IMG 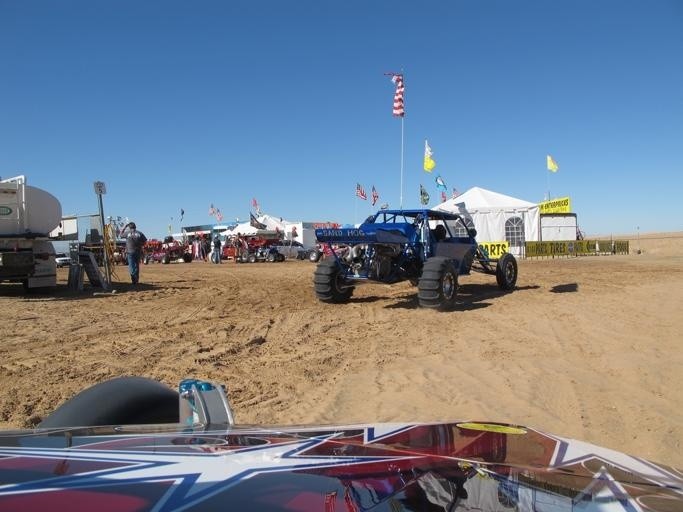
[418,186,540,257]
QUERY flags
[419,185,430,205]
[370,185,378,207]
[546,154,558,173]
[422,140,435,173]
[435,176,448,193]
[166,199,297,239]
[355,182,367,200]
[441,191,446,202]
[452,188,460,200]
[383,73,405,118]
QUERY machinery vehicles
[0,175,63,295]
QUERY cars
[54,252,71,268]
[0,376,681,510]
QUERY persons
[187,230,255,263]
[119,222,147,284]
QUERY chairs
[429,225,447,241]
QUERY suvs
[113,231,346,265]
[312,208,518,307]
[172,421,506,512]
